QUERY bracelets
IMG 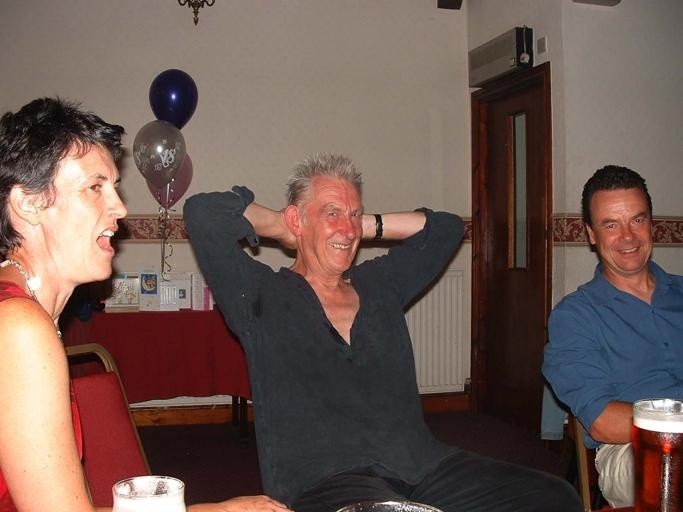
[372,212,385,243]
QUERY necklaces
[0,256,66,344]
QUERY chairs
[66,343,154,506]
[570,412,601,512]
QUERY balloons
[147,67,200,131]
[131,118,187,188]
[144,152,194,210]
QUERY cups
[633,397,683,512]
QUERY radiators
[405,269,464,395]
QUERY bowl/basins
[336,499,439,512]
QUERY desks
[66,310,253,447]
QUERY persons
[0,93,292,512]
[539,163,682,508]
[179,151,590,511]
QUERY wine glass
[110,477,184,512]
